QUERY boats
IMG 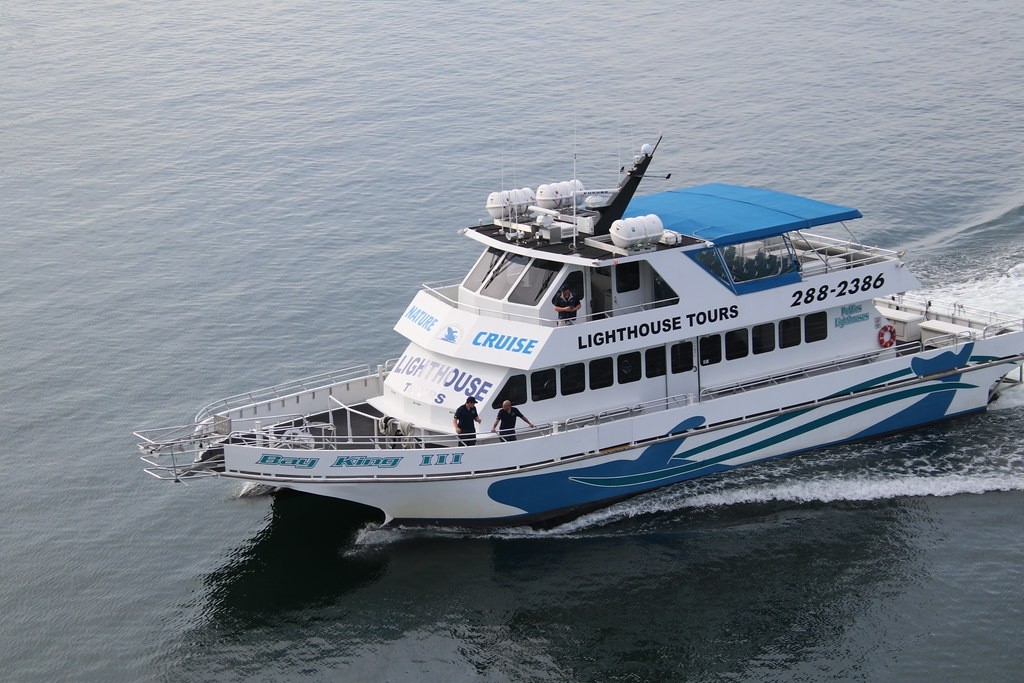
[130,116,1024,527]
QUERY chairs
[702,245,801,283]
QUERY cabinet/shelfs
[917,319,991,348]
[875,305,924,342]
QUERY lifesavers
[878,325,896,348]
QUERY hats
[467,396,478,403]
[560,284,569,291]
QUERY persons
[491,400,534,442]
[453,397,481,447]
[553,285,581,325]
